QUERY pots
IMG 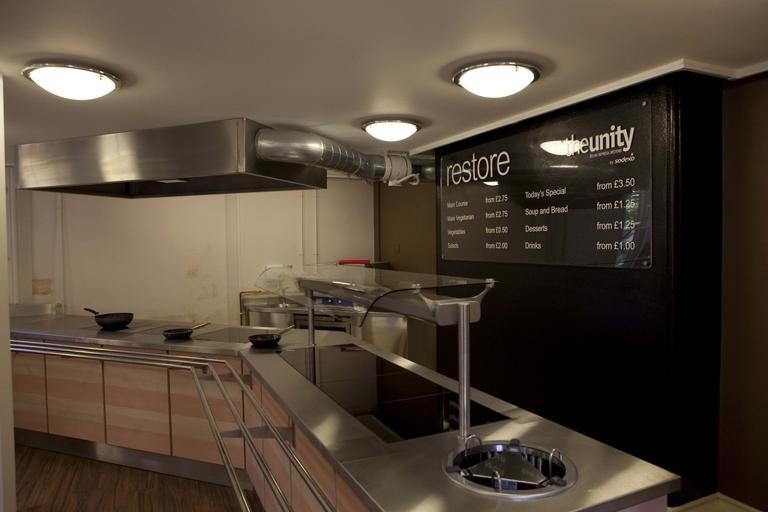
[83,307,294,347]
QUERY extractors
[15,119,327,199]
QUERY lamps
[540,138,584,158]
[20,59,125,103]
[447,59,546,101]
[361,117,422,143]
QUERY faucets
[281,286,290,307]
